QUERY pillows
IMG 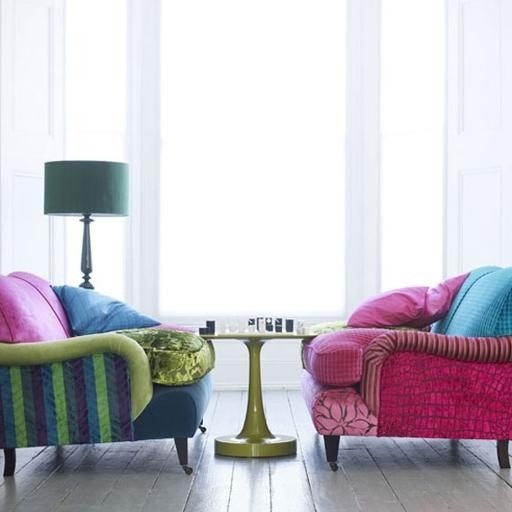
[348,271,470,326]
[49,286,161,334]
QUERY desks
[201,333,318,458]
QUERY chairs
[302,266,512,472]
[1,271,216,476]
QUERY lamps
[44,161,130,288]
